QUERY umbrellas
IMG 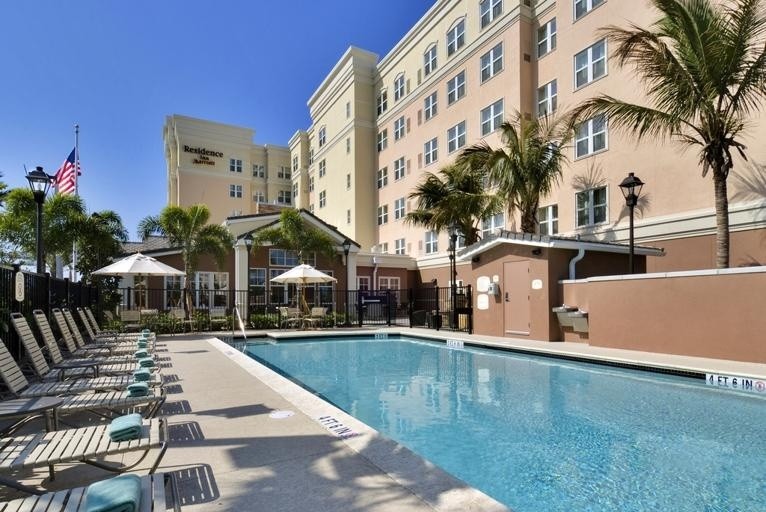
[268,263,338,310]
[90,248,187,323]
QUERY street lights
[24,166,53,274]
[342,238,353,327]
[617,173,646,273]
[447,233,458,330]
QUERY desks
[54,361,101,391]
[81,342,117,356]
[2,398,62,474]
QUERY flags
[52,146,81,197]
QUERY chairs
[2,477,181,512]
[51,308,156,353]
[11,311,162,380]
[2,387,167,426]
[275,307,328,331]
[2,415,169,496]
[1,340,164,414]
[32,309,158,363]
